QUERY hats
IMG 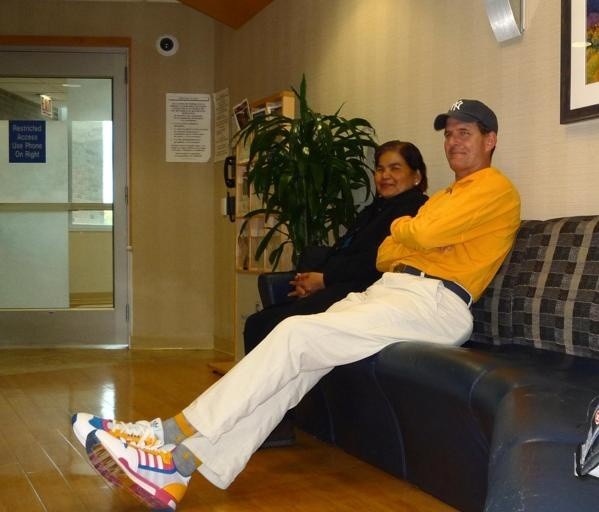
[433,97,499,138]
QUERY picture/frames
[562,0,597,122]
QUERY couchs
[237,217,597,512]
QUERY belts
[392,261,475,312]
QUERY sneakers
[85,428,195,511]
[69,411,177,452]
[256,426,298,450]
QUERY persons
[66,98,522,512]
[244,140,430,450]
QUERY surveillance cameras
[155,33,178,58]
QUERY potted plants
[229,72,381,309]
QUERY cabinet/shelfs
[234,91,299,274]
